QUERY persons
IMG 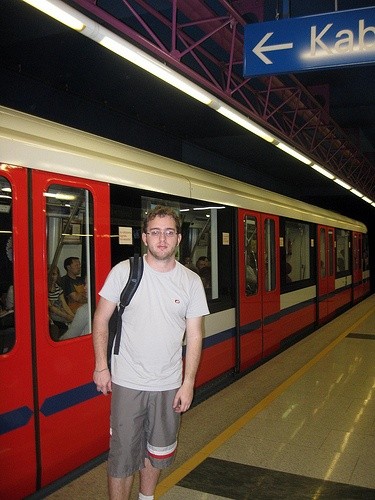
[244,236,258,296]
[91,205,211,500]
[177,255,211,312]
[55,256,83,306]
[58,284,90,340]
[48,264,75,342]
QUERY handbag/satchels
[107,254,143,361]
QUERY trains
[0,105,372,500]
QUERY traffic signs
[243,6,375,78]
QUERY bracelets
[95,368,108,372]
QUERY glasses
[145,230,178,237]
